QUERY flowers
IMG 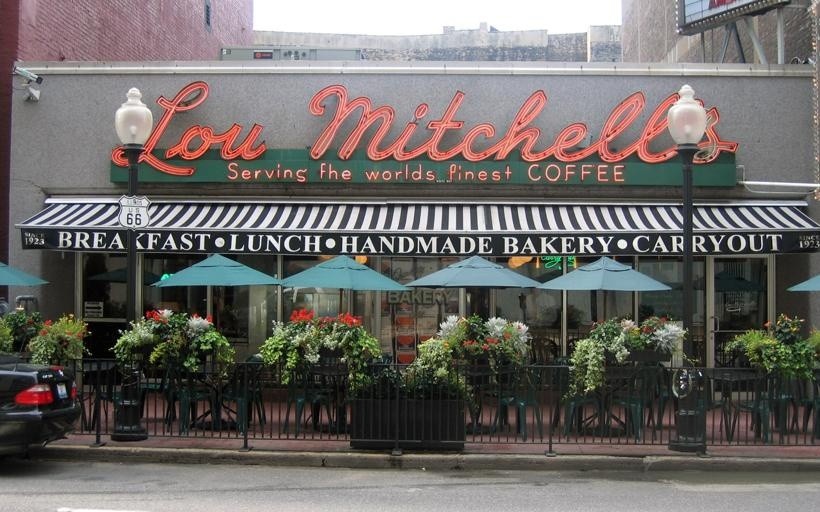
[0,310,44,353]
[403,313,533,377]
[24,313,93,369]
[107,310,236,377]
[254,309,383,385]
[561,314,689,401]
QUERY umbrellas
[534,253,673,321]
[0,260,50,287]
[279,248,414,312]
[405,253,545,320]
[149,255,283,361]
[788,273,820,292]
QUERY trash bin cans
[669,368,704,452]
[112,365,147,439]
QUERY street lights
[667,84,707,451]
[114,87,153,440]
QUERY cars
[0,352,82,463]
